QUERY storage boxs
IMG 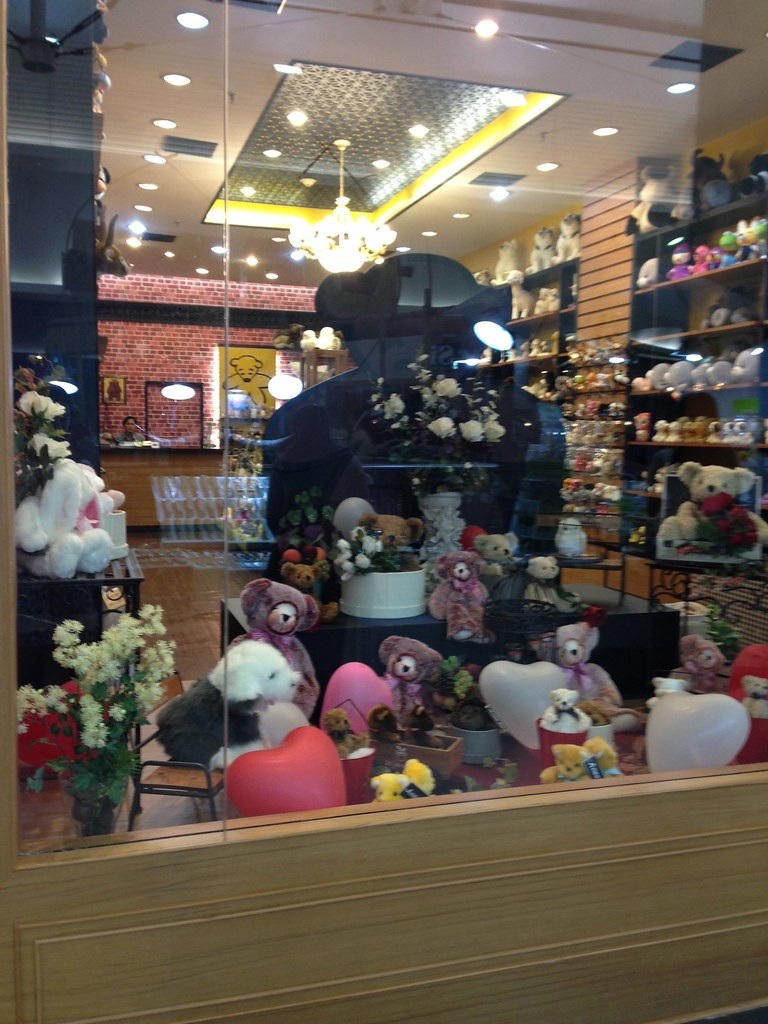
[379,732,462,780]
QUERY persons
[113,416,147,441]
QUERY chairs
[122,669,225,832]
[526,509,634,605]
[643,470,768,679]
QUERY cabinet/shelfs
[476,258,579,405]
[624,191,768,510]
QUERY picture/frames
[101,376,127,406]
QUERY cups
[106,510,129,559]
[449,723,501,764]
[535,717,587,769]
[586,723,613,747]
[341,746,380,806]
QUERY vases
[418,489,467,558]
[65,779,116,837]
[339,568,428,618]
[448,723,503,765]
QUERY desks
[17,549,148,812]
[219,582,681,728]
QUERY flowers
[542,580,605,629]
[13,352,73,504]
[694,493,759,560]
[16,603,180,806]
[331,522,408,581]
[365,344,506,499]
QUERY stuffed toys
[473,533,523,576]
[93,165,111,207]
[538,743,591,784]
[644,634,728,709]
[583,737,624,784]
[524,557,580,613]
[554,516,588,558]
[724,644,768,766]
[427,550,495,644]
[528,620,638,734]
[92,40,112,113]
[157,514,444,806]
[471,148,768,545]
[93,0,120,44]
[14,458,126,578]
[539,687,593,734]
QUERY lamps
[285,138,398,274]
